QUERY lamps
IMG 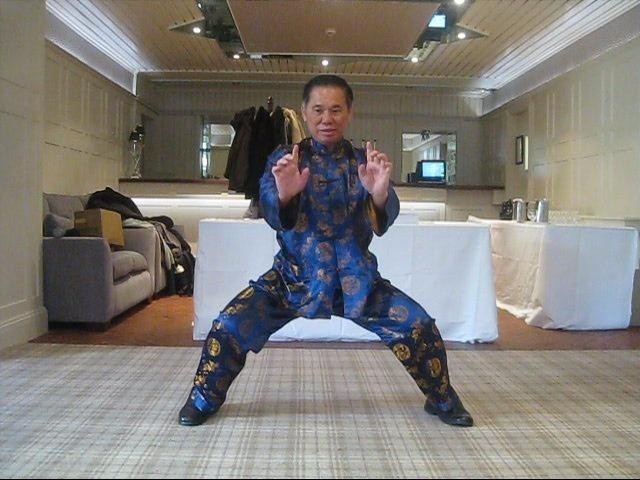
[128,123,145,180]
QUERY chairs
[43,191,185,333]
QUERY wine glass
[526,209,537,224]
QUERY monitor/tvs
[416,161,420,178]
[421,160,446,181]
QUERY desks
[467,215,637,332]
[193,219,499,344]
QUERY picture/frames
[514,134,524,165]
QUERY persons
[177,74,475,427]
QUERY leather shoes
[424,398,473,426]
[178,398,210,426]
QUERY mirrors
[197,112,236,178]
[399,131,457,185]
